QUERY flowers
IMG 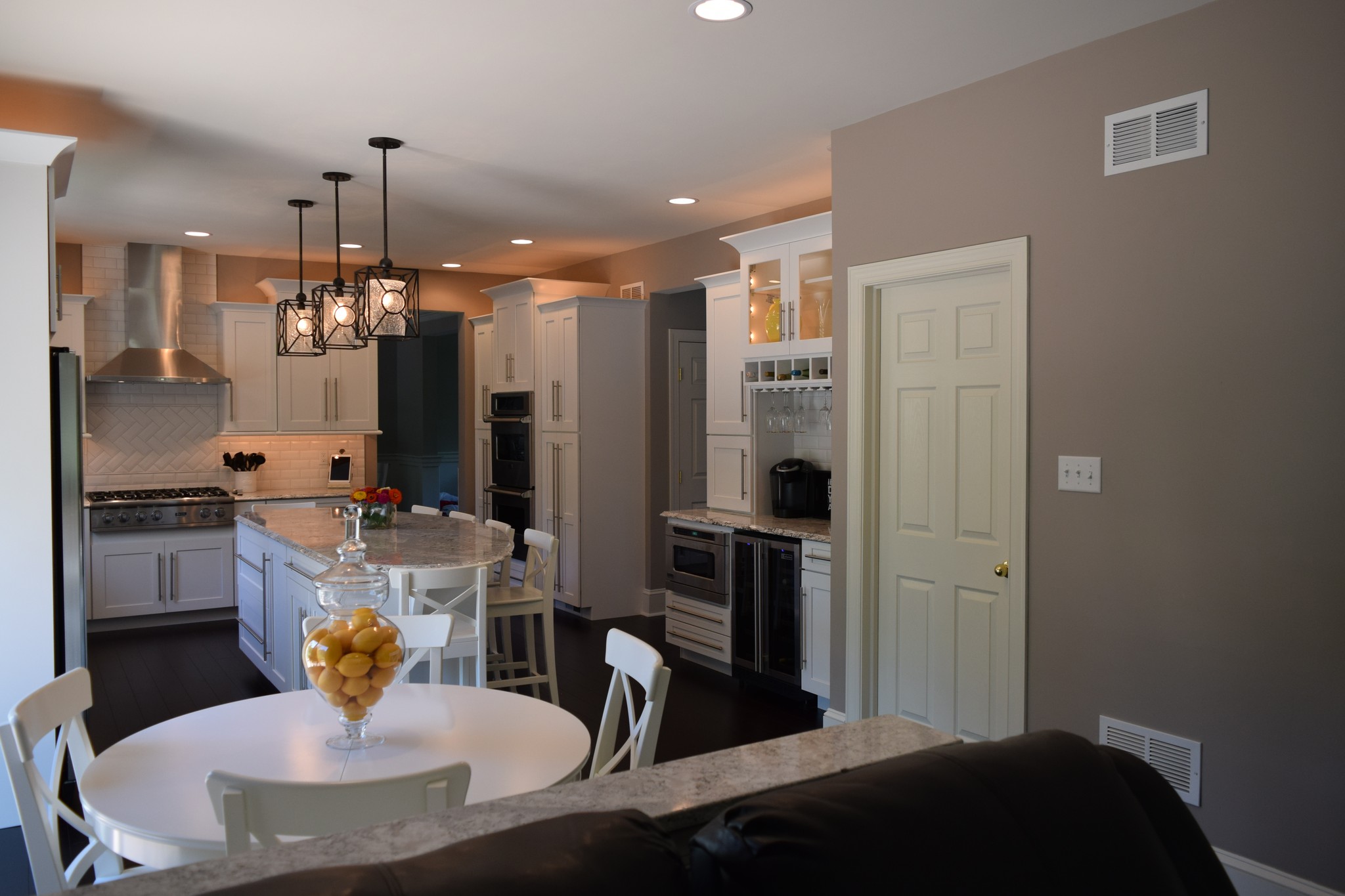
[349,486,402,525]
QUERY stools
[410,505,560,706]
[389,561,494,689]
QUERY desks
[79,685,591,869]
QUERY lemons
[303,607,401,721]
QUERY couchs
[205,730,1238,896]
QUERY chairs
[0,667,153,896]
[204,762,470,856]
[589,629,671,779]
[302,614,454,685]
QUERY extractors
[85,242,231,384]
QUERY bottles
[746,372,758,378]
[819,369,828,375]
[765,299,781,342]
[791,369,809,378]
[777,373,792,381]
[765,372,775,377]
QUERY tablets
[328,454,352,483]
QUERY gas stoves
[86,487,235,532]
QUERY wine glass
[765,392,778,433]
[815,299,830,338]
[818,390,829,424]
[827,390,831,431]
[777,392,793,432]
[793,389,808,433]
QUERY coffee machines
[769,458,813,518]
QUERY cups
[233,471,257,492]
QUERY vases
[357,502,397,529]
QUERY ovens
[483,392,535,490]
[665,523,729,605]
[482,484,535,561]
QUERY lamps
[312,172,368,350]
[277,199,326,357]
[355,137,420,341]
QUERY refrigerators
[48,345,88,678]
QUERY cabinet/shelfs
[267,293,378,431]
[236,521,326,692]
[739,232,833,358]
[799,536,830,700]
[92,533,235,619]
[217,311,277,432]
[706,282,756,514]
[666,590,731,664]
[474,322,493,521]
[540,306,644,610]
[493,292,542,393]
